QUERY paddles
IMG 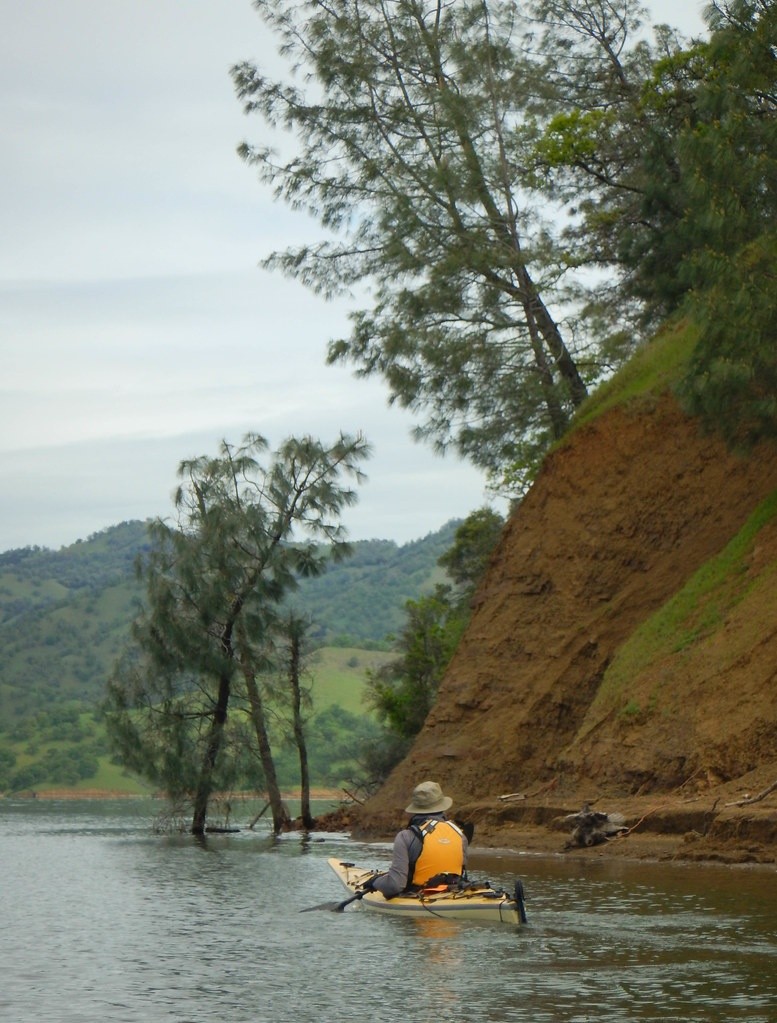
[298,822,476,914]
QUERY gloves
[363,874,379,893]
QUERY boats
[328,857,529,927]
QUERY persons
[364,781,469,898]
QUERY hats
[405,782,454,814]
